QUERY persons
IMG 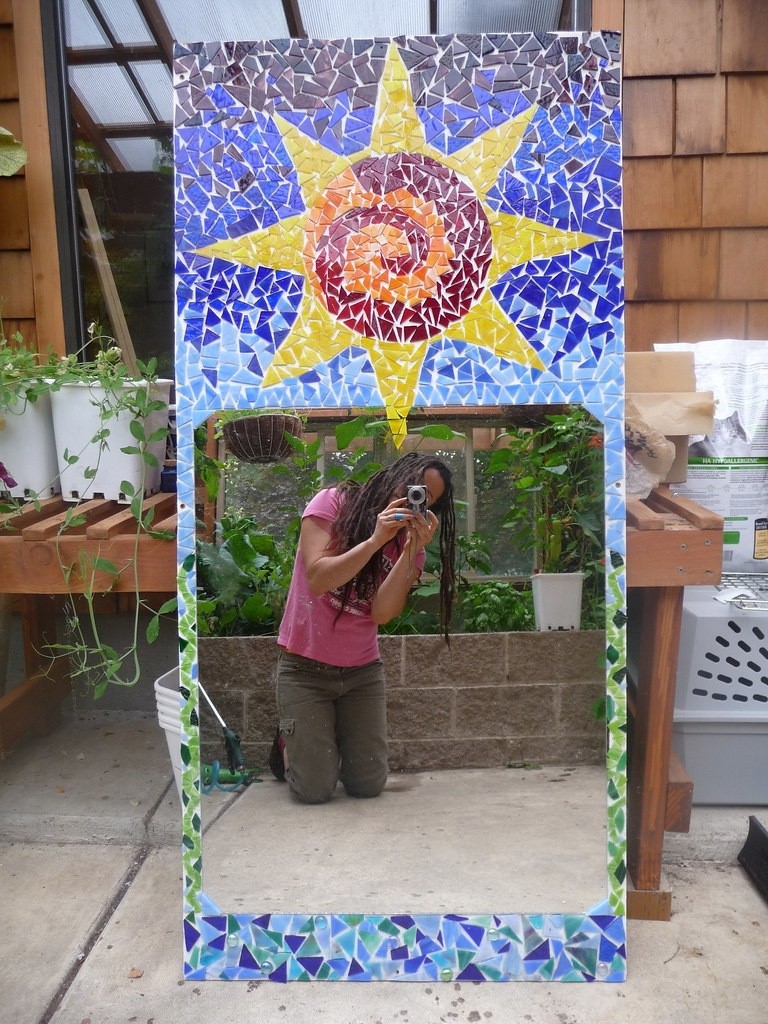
[266,452,460,804]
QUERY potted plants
[215,410,306,465]
[486,406,603,632]
[0,320,177,699]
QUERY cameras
[406,485,427,523]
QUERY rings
[396,513,404,521]
[388,514,392,521]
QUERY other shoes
[269,727,286,782]
